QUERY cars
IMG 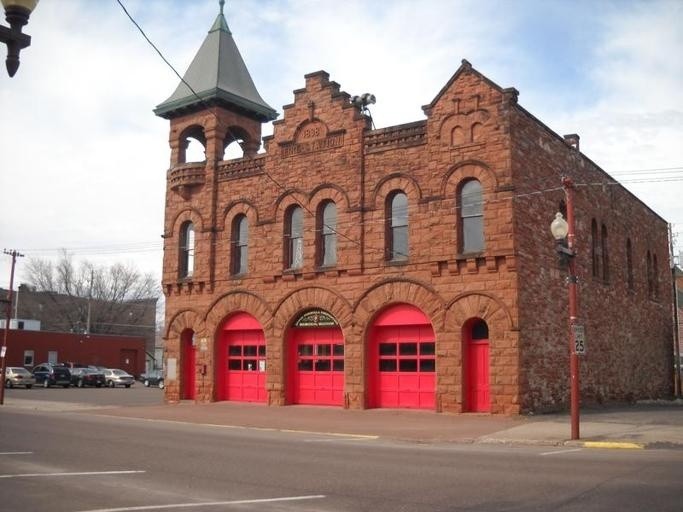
[5,362,136,390]
[138,370,164,389]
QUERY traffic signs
[571,323,586,357]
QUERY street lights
[549,177,590,441]
